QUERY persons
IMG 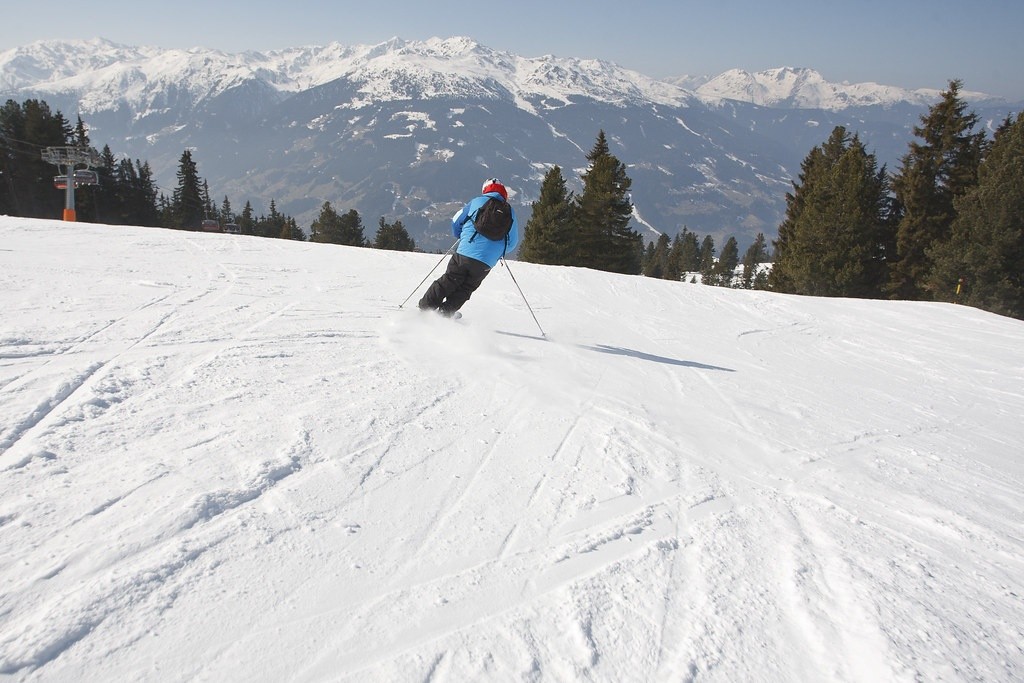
[418,178,519,319]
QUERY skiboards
[418,305,462,320]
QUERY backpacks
[464,197,513,258]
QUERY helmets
[481,177,508,201]
